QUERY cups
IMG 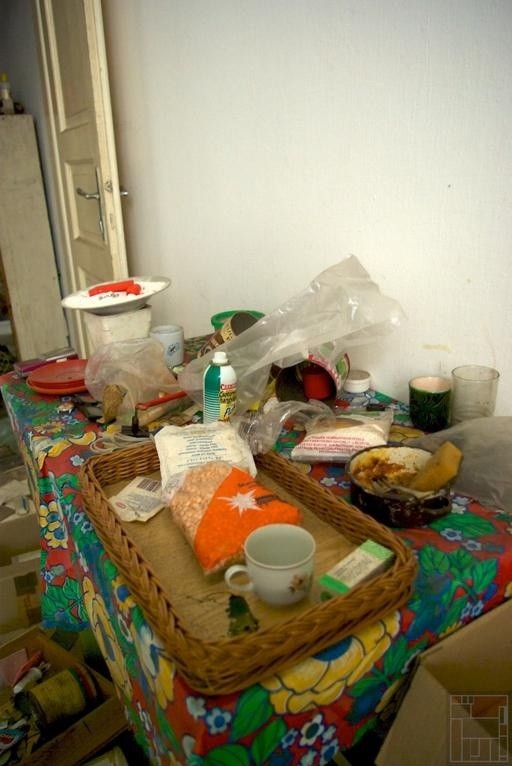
[223,523,317,606]
[304,366,332,401]
[409,366,499,433]
[149,323,187,369]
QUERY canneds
[24,662,99,729]
[197,311,258,360]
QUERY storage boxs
[1,464,138,765]
[378,599,510,765]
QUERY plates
[27,360,97,392]
[60,278,171,316]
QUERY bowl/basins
[344,444,454,528]
[211,310,266,330]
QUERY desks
[1,332,512,766]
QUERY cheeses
[409,441,462,493]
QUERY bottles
[202,352,236,425]
[1,72,17,116]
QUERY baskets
[77,430,418,696]
